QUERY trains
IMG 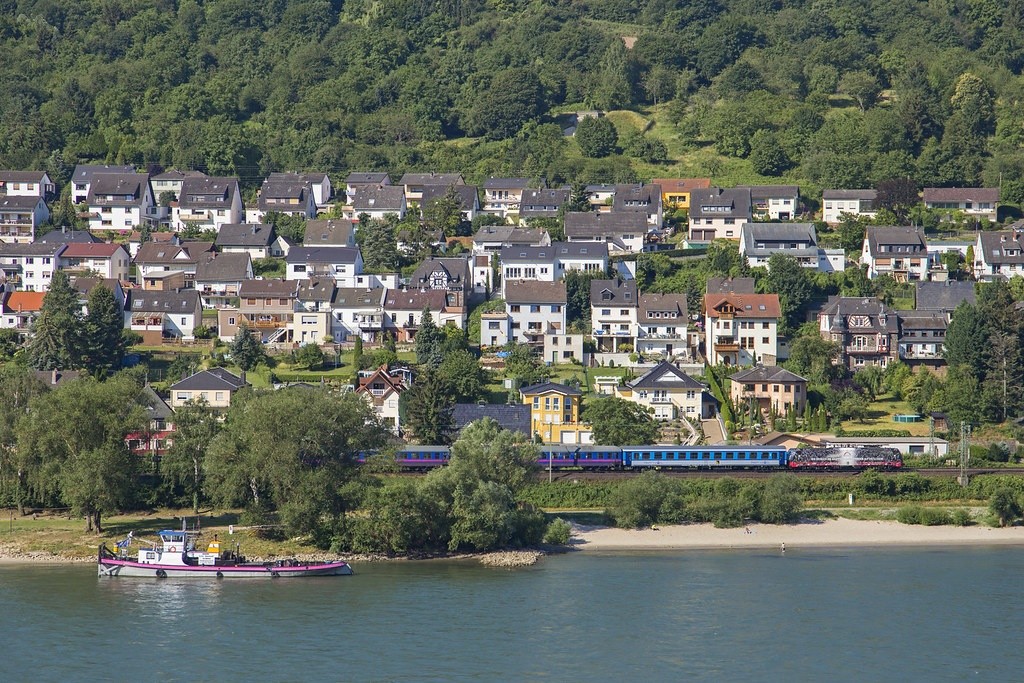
[349,444,903,472]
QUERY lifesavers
[156,568,165,577]
[170,546,176,552]
[271,571,278,577]
[217,570,222,576]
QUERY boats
[98,530,354,578]
